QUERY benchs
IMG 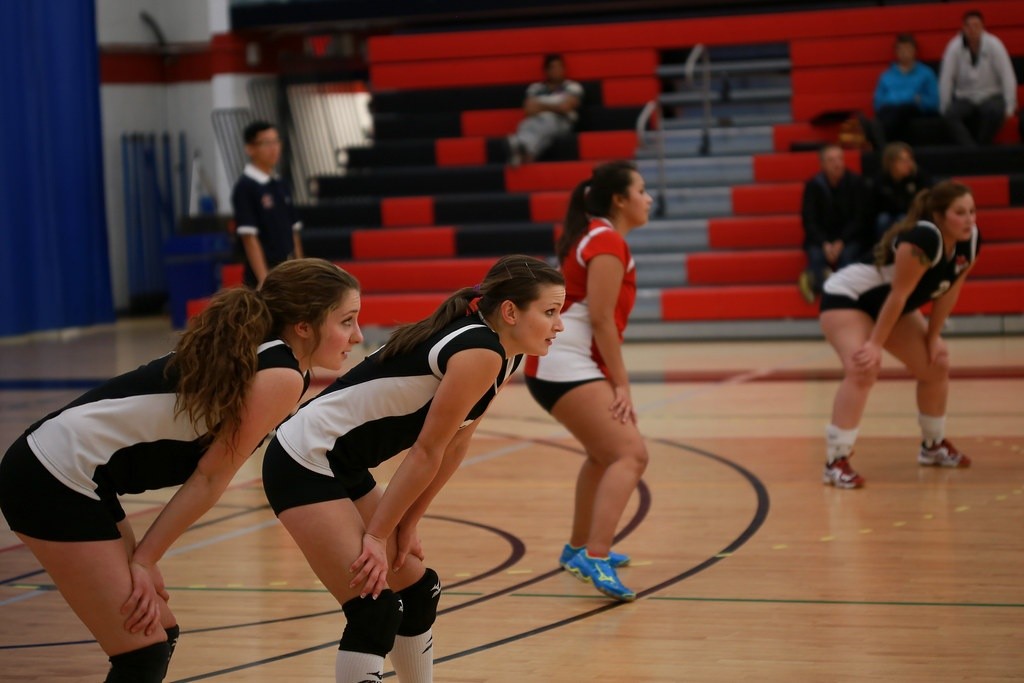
[186,0,1024,331]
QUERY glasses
[253,137,282,148]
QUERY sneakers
[919,439,971,468]
[559,541,637,603]
[822,455,867,489]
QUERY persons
[262,254,566,682]
[819,182,983,489]
[507,55,583,164]
[0,257,363,683]
[524,160,653,602]
[229,120,303,290]
[800,11,1016,294]
